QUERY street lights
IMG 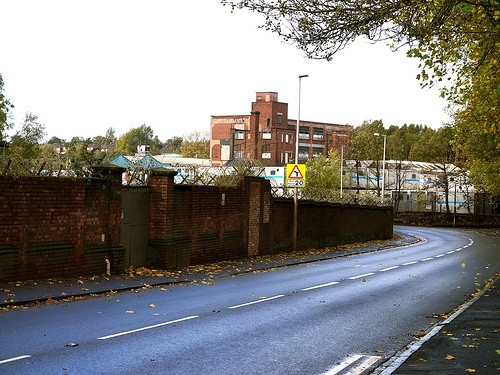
[373,133,387,203]
[292,74,308,252]
